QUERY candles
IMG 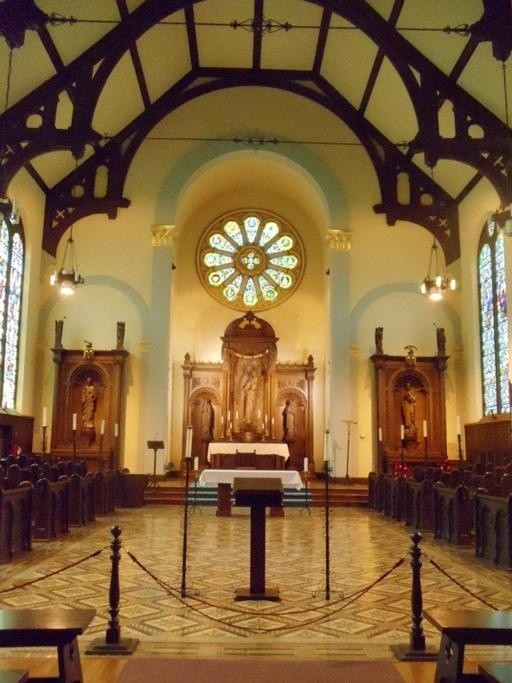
[185,427,193,457]
[401,425,404,439]
[304,457,308,473]
[379,428,382,441]
[423,420,427,437]
[42,407,47,426]
[101,420,105,435]
[194,456,199,470]
[323,434,329,461]
[115,424,118,436]
[73,413,77,430]
[457,416,460,434]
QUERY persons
[80,375,96,430]
[399,379,417,435]
[282,398,296,441]
[238,364,259,423]
[198,396,213,441]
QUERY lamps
[50,160,84,295]
[421,165,457,301]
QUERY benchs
[0,608,96,683]
[423,609,512,683]
